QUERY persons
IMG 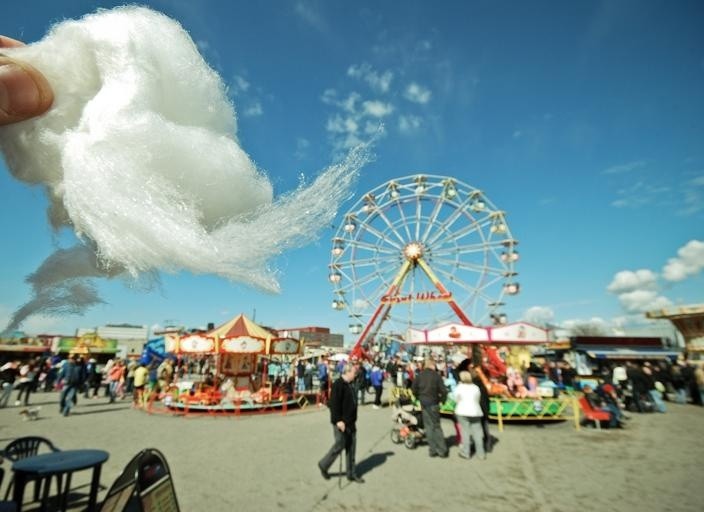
[264,353,367,403]
[318,363,366,483]
[0,34,56,128]
[368,353,583,409]
[583,353,704,431]
[0,353,163,419]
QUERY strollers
[387,387,426,450]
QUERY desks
[12,449,109,512]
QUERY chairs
[4,436,63,502]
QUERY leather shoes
[348,476,364,483]
[318,463,330,479]
